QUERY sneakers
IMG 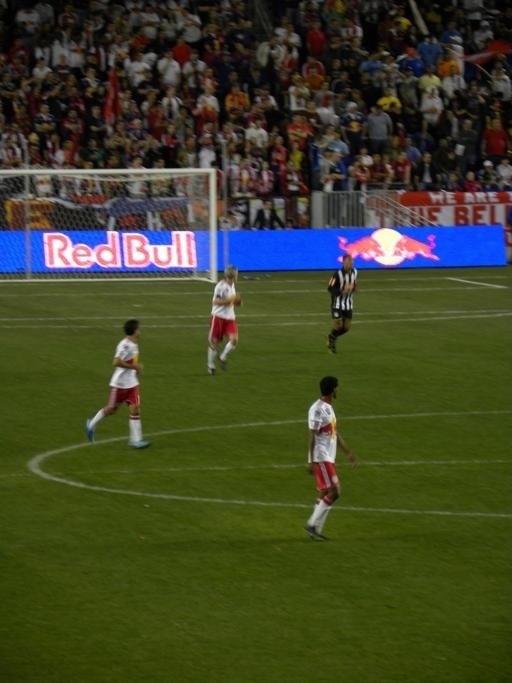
[327,333,337,353]
[303,524,325,540]
[87,419,94,442]
[128,440,149,449]
[208,362,216,375]
[217,357,228,372]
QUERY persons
[208,264,242,374]
[327,253,358,352]
[304,375,354,539]
[2,1,512,230]
[86,319,151,447]
